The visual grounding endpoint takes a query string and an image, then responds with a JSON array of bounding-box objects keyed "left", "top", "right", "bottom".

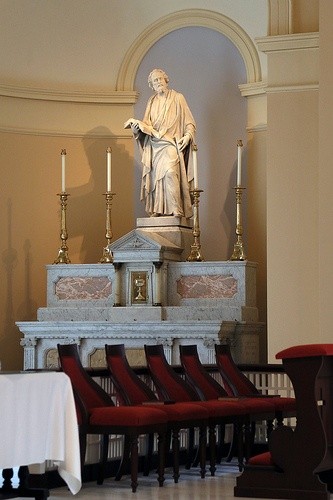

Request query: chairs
[
  {"left": 214, "top": 346, "right": 296, "bottom": 463},
  {"left": 143, "top": 344, "right": 253, "bottom": 476},
  {"left": 179, "top": 345, "right": 278, "bottom": 472},
  {"left": 57, "top": 343, "right": 166, "bottom": 492},
  {"left": 105, "top": 344, "right": 209, "bottom": 483}
]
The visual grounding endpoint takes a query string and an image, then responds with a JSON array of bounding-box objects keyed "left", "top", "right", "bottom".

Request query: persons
[{"left": 124, "top": 69, "right": 196, "bottom": 218}]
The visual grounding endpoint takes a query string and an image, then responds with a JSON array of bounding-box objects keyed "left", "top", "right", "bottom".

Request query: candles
[
  {"left": 60, "top": 149, "right": 67, "bottom": 192},
  {"left": 192, "top": 145, "right": 199, "bottom": 190},
  {"left": 236, "top": 139, "right": 243, "bottom": 186},
  {"left": 106, "top": 147, "right": 113, "bottom": 192}
]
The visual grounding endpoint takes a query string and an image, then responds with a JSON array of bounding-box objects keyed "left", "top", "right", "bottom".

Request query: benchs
[{"left": 234, "top": 344, "right": 333, "bottom": 500}]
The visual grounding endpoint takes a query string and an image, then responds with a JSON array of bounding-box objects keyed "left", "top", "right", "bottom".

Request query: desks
[
  {"left": 0, "top": 374, "right": 81, "bottom": 495},
  {"left": 13, "top": 318, "right": 237, "bottom": 375}
]
